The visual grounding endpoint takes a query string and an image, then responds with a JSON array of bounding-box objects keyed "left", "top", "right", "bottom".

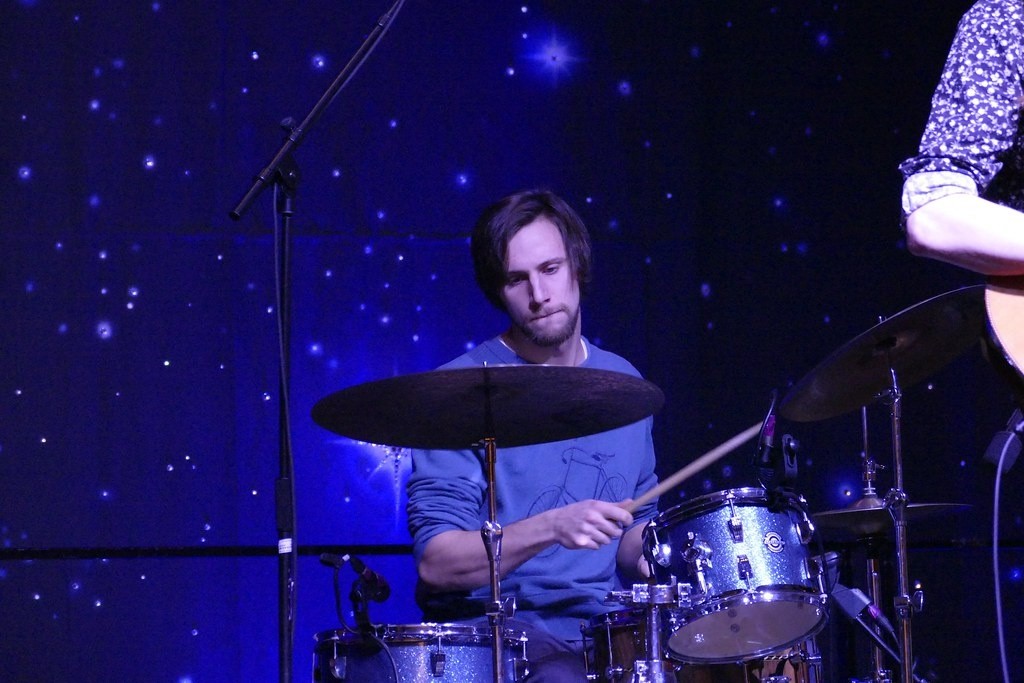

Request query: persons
[
  {"left": 897, "top": 0, "right": 1024, "bottom": 284},
  {"left": 406, "top": 188, "right": 661, "bottom": 683}
]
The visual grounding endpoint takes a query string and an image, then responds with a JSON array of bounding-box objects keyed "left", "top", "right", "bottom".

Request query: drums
[
  {"left": 642, "top": 486, "right": 825, "bottom": 664},
  {"left": 576, "top": 606, "right": 713, "bottom": 683},
  {"left": 313, "top": 623, "right": 529, "bottom": 683}
]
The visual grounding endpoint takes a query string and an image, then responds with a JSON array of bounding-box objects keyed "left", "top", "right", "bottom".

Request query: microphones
[
  {"left": 754, "top": 393, "right": 778, "bottom": 488},
  {"left": 351, "top": 555, "right": 391, "bottom": 602}
]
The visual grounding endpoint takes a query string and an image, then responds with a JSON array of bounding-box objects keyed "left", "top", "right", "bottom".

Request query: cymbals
[
  {"left": 779, "top": 284, "right": 985, "bottom": 423},
  {"left": 312, "top": 364, "right": 665, "bottom": 452},
  {"left": 809, "top": 498, "right": 966, "bottom": 535}
]
